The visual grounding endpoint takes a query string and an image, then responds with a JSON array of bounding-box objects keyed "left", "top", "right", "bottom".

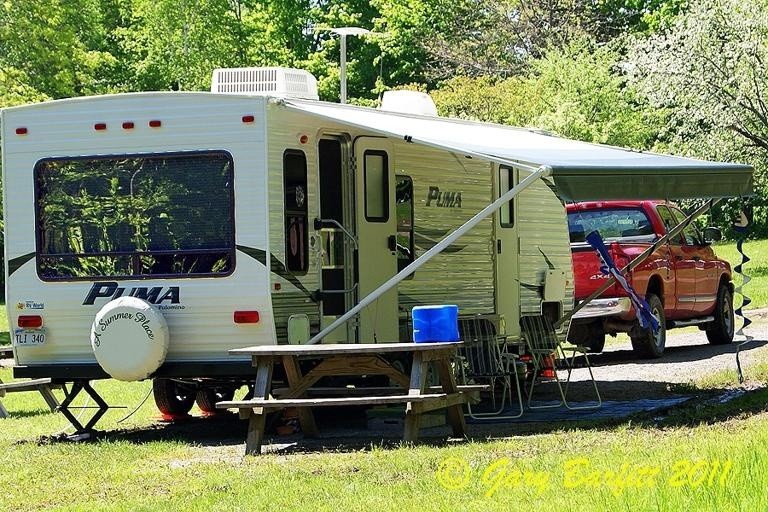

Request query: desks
[{"left": 229, "top": 340, "right": 481, "bottom": 456}]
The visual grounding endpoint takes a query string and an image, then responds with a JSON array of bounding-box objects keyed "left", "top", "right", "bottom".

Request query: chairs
[
  {"left": 569, "top": 224, "right": 585, "bottom": 242},
  {"left": 622, "top": 229, "right": 643, "bottom": 237},
  {"left": 519, "top": 315, "right": 602, "bottom": 411},
  {"left": 456, "top": 318, "right": 524, "bottom": 420}
]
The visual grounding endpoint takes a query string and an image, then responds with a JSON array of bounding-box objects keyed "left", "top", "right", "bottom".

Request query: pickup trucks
[{"left": 566, "top": 200, "right": 735, "bottom": 358}]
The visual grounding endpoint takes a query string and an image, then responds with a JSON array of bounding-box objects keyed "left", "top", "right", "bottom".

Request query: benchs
[
  {"left": 214, "top": 393, "right": 446, "bottom": 410},
  {"left": 272, "top": 384, "right": 492, "bottom": 398},
  {"left": 0, "top": 378, "right": 61, "bottom": 418}
]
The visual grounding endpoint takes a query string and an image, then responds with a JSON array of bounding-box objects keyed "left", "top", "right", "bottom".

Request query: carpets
[{"left": 460, "top": 396, "right": 695, "bottom": 424}]
